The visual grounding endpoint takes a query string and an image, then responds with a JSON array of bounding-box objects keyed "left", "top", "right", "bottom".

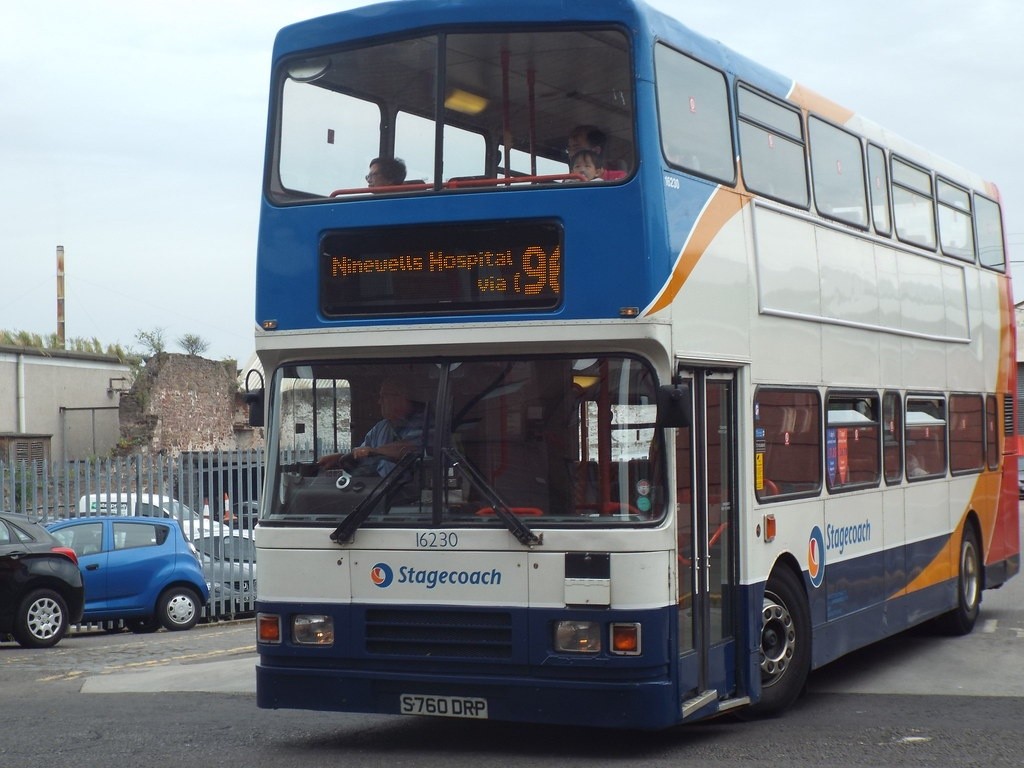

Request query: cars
[
  {"left": 1, "top": 509, "right": 85, "bottom": 649},
  {"left": 215, "top": 501, "right": 260, "bottom": 527},
  {"left": 32, "top": 515, "right": 210, "bottom": 633},
  {"left": 187, "top": 528, "right": 256, "bottom": 614}
]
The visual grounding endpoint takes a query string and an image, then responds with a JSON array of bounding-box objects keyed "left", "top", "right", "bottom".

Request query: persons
[
  {"left": 562, "top": 124, "right": 629, "bottom": 183},
  {"left": 316, "top": 375, "right": 435, "bottom": 507},
  {"left": 365, "top": 158, "right": 406, "bottom": 195},
  {"left": 906, "top": 452, "right": 930, "bottom": 477}
]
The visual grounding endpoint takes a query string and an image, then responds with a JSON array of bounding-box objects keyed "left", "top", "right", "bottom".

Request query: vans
[{"left": 78, "top": 493, "right": 231, "bottom": 553}]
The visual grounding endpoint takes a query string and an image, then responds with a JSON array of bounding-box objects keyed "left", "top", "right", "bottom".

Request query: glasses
[
  {"left": 365, "top": 171, "right": 383, "bottom": 180},
  {"left": 565, "top": 145, "right": 593, "bottom": 154}
]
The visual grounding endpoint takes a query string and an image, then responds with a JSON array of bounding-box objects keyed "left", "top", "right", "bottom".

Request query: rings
[{"left": 361, "top": 450, "right": 365, "bottom": 452}]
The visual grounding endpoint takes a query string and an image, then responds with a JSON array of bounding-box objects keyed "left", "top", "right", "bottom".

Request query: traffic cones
[
  {"left": 225, "top": 492, "right": 238, "bottom": 524},
  {"left": 203, "top": 497, "right": 211, "bottom": 518}
]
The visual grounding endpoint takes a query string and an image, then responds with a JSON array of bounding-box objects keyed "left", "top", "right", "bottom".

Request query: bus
[{"left": 240, "top": 0, "right": 1022, "bottom": 737}]
